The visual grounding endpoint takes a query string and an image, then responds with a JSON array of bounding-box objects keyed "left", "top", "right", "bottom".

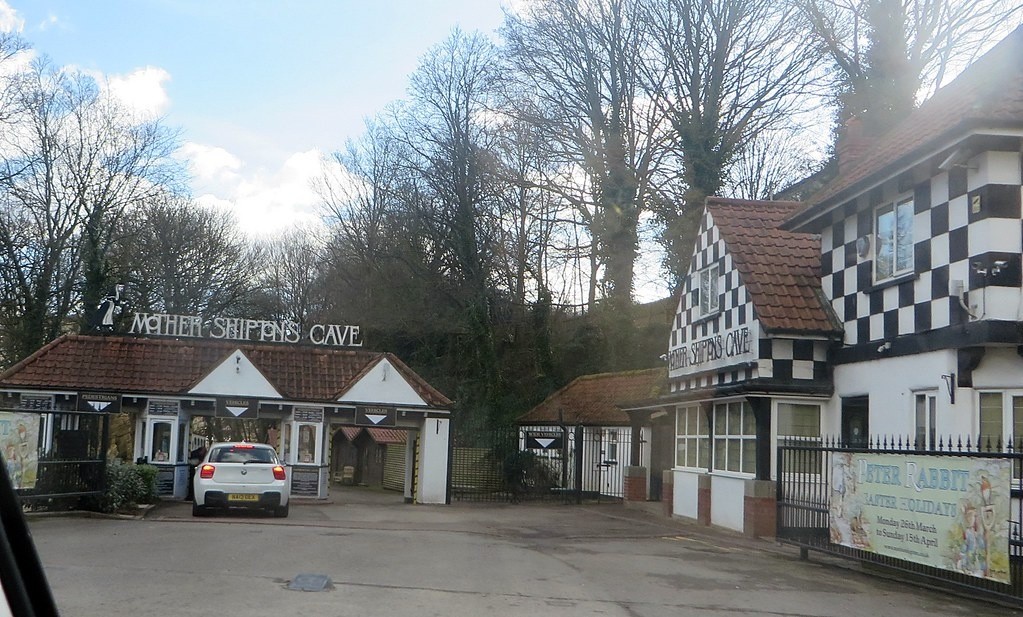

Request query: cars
[{"left": 191, "top": 442, "right": 290, "bottom": 519}]
